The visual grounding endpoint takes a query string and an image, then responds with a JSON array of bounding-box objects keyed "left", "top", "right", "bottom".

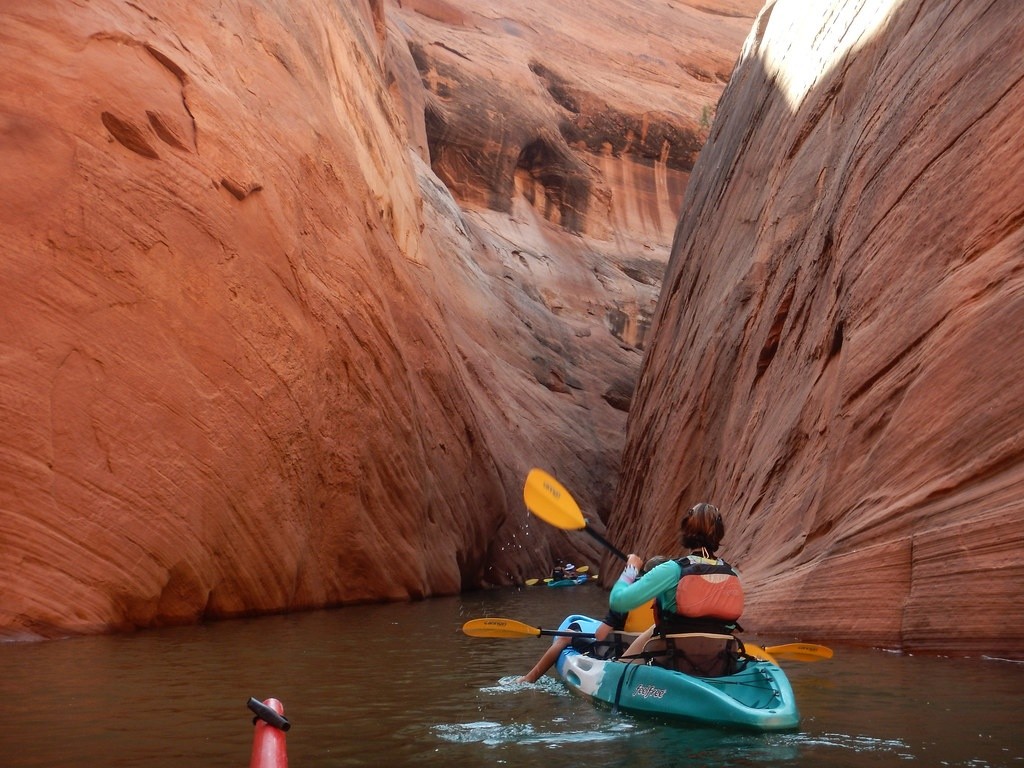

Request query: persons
[
  {"left": 513, "top": 502, "right": 747, "bottom": 685},
  {"left": 565, "top": 563, "right": 576, "bottom": 579},
  {"left": 550, "top": 557, "right": 570, "bottom": 579}
]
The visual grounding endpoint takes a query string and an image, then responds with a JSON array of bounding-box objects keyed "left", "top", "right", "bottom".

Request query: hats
[
  {"left": 644, "top": 555, "right": 668, "bottom": 573},
  {"left": 554, "top": 558, "right": 563, "bottom": 567},
  {"left": 564, "top": 563, "right": 574, "bottom": 571}
]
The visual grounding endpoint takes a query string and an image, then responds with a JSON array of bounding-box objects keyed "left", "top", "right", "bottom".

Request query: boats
[
  {"left": 546, "top": 573, "right": 589, "bottom": 588},
  {"left": 552, "top": 615, "right": 803, "bottom": 729}
]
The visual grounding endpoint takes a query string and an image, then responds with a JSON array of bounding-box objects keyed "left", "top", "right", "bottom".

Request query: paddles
[
  {"left": 524, "top": 466, "right": 782, "bottom": 671},
  {"left": 525, "top": 565, "right": 599, "bottom": 586},
  {"left": 462, "top": 617, "right": 834, "bottom": 663}
]
